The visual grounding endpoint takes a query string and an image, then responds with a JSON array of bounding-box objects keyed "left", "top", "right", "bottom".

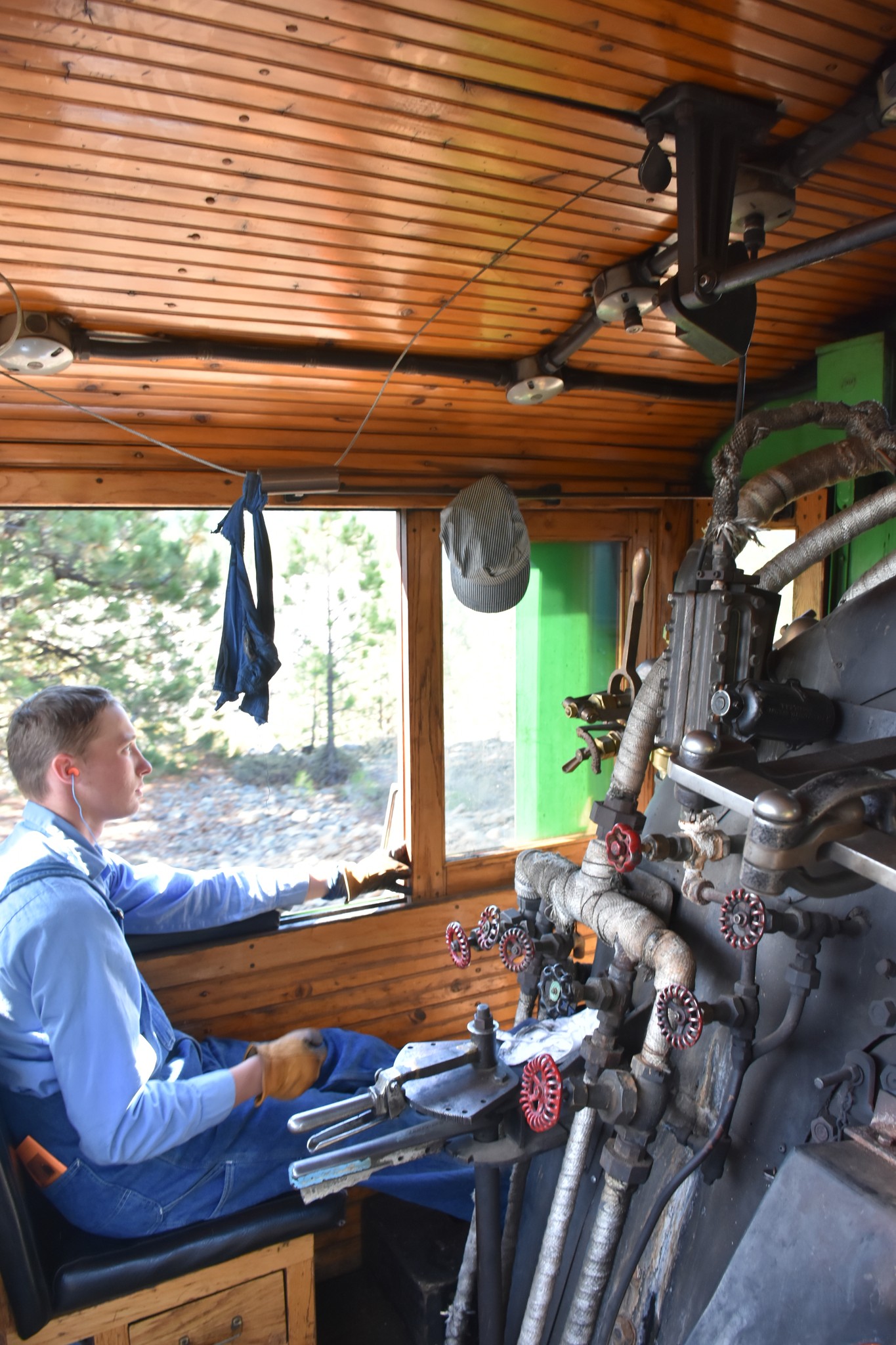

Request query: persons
[{"left": 0, "top": 687, "right": 508, "bottom": 1236}]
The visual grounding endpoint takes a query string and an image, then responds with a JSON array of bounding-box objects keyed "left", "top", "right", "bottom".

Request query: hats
[{"left": 439, "top": 472, "right": 532, "bottom": 615}]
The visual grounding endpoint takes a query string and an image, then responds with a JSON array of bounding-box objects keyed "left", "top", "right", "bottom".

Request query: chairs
[{"left": 0, "top": 1118, "right": 346, "bottom": 1345}]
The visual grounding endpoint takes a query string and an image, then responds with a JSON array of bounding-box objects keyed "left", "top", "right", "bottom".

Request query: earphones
[{"left": 68, "top": 768, "right": 79, "bottom": 776}]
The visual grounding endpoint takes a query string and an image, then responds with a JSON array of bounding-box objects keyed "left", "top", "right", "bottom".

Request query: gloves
[
  {"left": 341, "top": 840, "right": 413, "bottom": 905},
  {"left": 243, "top": 1027, "right": 328, "bottom": 1109}
]
